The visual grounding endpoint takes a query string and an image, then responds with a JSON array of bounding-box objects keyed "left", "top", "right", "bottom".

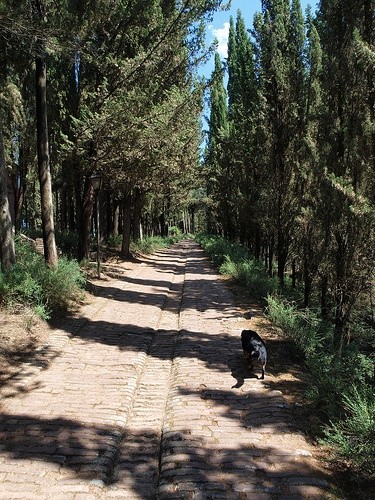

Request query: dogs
[{"left": 240, "top": 329, "right": 266, "bottom": 380}]
